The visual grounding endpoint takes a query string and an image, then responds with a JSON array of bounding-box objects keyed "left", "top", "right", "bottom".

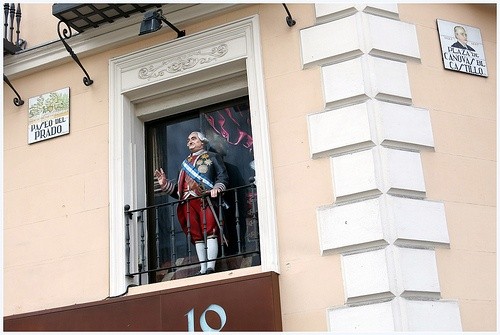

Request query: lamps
[{"left": 137, "top": 8, "right": 186, "bottom": 38}]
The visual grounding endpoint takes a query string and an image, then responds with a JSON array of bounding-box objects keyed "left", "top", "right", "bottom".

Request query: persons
[
  {"left": 451, "top": 26, "right": 475, "bottom": 51},
  {"left": 154, "top": 132, "right": 229, "bottom": 275}
]
